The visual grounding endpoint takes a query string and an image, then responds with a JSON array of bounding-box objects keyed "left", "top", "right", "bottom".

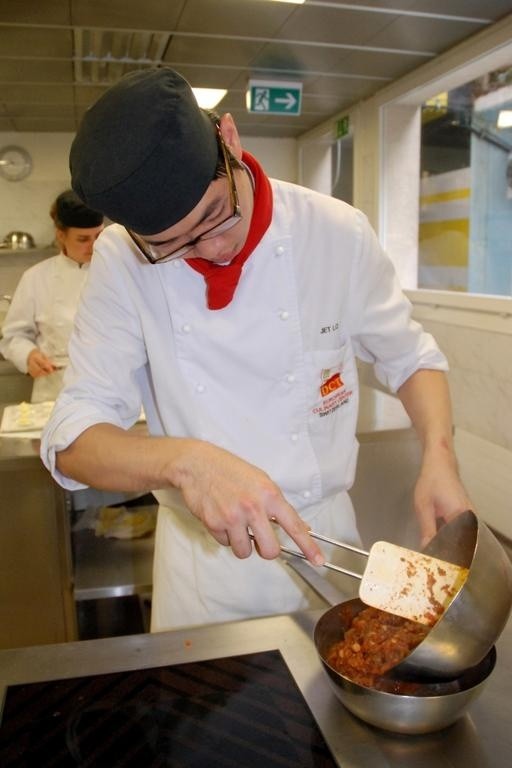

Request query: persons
[
  {"left": 0, "top": 190, "right": 105, "bottom": 403},
  {"left": 40, "top": 66, "right": 476, "bottom": 634}
]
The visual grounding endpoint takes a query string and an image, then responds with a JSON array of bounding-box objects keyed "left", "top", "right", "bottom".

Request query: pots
[
  {"left": 398, "top": 510, "right": 510, "bottom": 677},
  {"left": 286, "top": 555, "right": 497, "bottom": 735}
]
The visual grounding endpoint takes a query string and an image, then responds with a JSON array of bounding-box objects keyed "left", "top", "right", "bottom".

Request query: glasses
[{"left": 125, "top": 122, "right": 243, "bottom": 264}]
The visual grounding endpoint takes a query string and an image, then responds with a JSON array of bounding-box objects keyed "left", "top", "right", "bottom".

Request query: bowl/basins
[{"left": 5, "top": 233, "right": 36, "bottom": 246}]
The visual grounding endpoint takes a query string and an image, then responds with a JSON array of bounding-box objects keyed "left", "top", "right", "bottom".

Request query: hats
[
  {"left": 54, "top": 190, "right": 104, "bottom": 228},
  {"left": 68, "top": 63, "right": 219, "bottom": 236}
]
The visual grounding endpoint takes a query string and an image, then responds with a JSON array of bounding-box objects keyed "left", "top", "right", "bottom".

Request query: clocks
[{"left": 0, "top": 145, "right": 31, "bottom": 182}]
